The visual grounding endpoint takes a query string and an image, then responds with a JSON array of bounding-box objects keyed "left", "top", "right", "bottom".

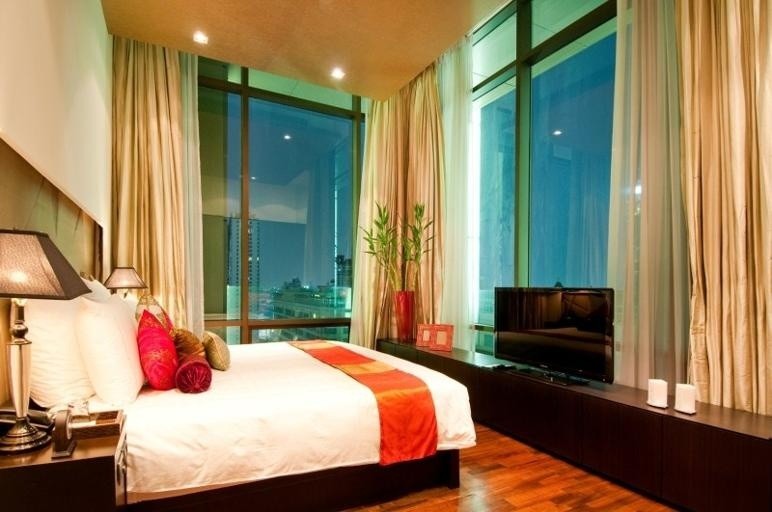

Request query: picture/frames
[
  {"left": 428, "top": 324, "right": 455, "bottom": 353},
  {"left": 415, "top": 321, "right": 432, "bottom": 346}
]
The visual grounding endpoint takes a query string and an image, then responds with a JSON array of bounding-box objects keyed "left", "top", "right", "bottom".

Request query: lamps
[
  {"left": 103, "top": 266, "right": 148, "bottom": 300},
  {"left": 0, "top": 223, "right": 92, "bottom": 452}
]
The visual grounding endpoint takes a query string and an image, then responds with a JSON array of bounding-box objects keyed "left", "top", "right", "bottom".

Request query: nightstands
[{"left": 1, "top": 412, "right": 132, "bottom": 512}]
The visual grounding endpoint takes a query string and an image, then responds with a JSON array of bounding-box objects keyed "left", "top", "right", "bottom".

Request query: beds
[{"left": 1, "top": 327, "right": 478, "bottom": 511}]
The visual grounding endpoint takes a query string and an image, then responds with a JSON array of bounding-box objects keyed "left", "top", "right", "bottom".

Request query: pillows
[{"left": 22, "top": 276, "right": 231, "bottom": 411}]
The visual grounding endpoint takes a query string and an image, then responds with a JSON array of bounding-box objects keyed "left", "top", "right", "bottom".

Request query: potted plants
[{"left": 358, "top": 198, "right": 438, "bottom": 344}]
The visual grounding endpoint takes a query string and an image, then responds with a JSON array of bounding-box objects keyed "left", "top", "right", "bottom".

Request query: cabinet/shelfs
[{"left": 371, "top": 335, "right": 772, "bottom": 512}]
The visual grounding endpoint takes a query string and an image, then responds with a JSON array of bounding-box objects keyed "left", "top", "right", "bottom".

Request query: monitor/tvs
[{"left": 494, "top": 287, "right": 614, "bottom": 387}]
